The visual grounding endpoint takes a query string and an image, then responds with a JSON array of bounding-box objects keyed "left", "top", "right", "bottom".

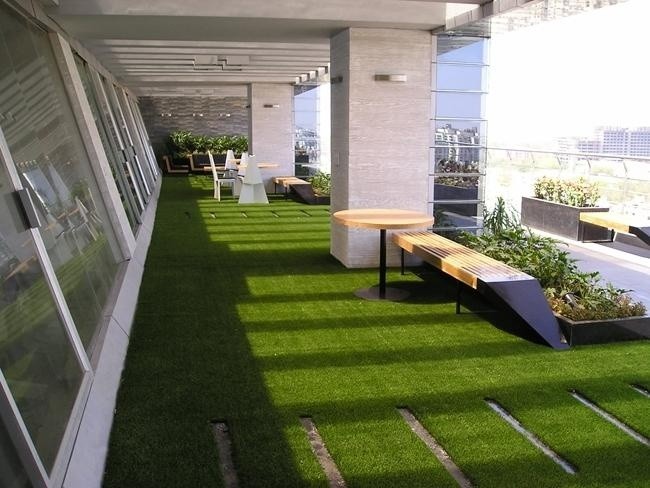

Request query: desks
[
  {"left": 233, "top": 162, "right": 280, "bottom": 190},
  {"left": 331, "top": 207, "right": 435, "bottom": 303}
]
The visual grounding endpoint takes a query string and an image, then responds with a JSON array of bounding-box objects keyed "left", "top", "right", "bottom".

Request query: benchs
[
  {"left": 392, "top": 230, "right": 570, "bottom": 354},
  {"left": 272, "top": 176, "right": 313, "bottom": 204}
]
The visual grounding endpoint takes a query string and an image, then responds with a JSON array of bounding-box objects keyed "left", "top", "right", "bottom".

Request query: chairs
[{"left": 162, "top": 149, "right": 239, "bottom": 202}]
